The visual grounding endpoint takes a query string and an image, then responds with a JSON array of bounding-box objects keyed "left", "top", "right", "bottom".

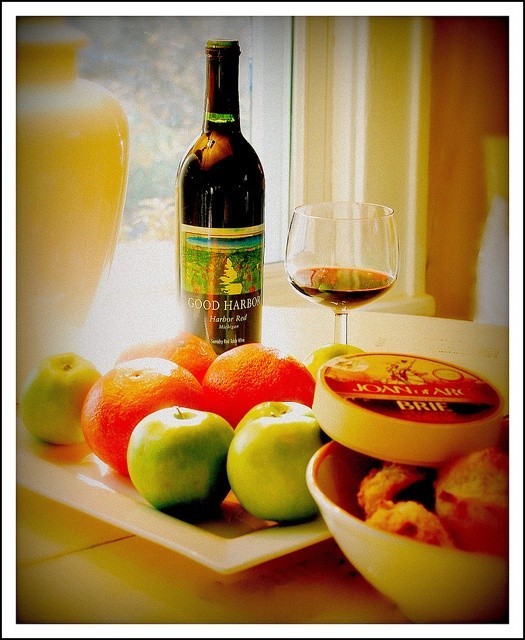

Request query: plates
[{"left": 16, "top": 304, "right": 508, "bottom": 575}]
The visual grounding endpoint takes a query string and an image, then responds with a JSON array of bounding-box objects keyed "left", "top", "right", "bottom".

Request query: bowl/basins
[{"left": 304, "top": 437, "right": 509, "bottom": 623}]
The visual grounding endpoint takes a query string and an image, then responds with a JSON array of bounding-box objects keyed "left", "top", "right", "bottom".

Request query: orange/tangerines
[
  {"left": 205, "top": 340, "right": 317, "bottom": 427},
  {"left": 80, "top": 357, "right": 205, "bottom": 475},
  {"left": 110, "top": 329, "right": 215, "bottom": 379}
]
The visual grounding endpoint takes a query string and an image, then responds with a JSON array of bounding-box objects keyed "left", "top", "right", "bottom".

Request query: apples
[
  {"left": 125, "top": 404, "right": 240, "bottom": 514},
  {"left": 222, "top": 398, "right": 325, "bottom": 519},
  {"left": 19, "top": 349, "right": 104, "bottom": 444}
]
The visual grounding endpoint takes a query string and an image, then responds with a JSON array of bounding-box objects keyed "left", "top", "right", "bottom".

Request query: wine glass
[{"left": 285, "top": 202, "right": 399, "bottom": 345}]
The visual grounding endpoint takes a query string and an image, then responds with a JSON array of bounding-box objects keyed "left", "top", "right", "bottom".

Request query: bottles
[{"left": 176, "top": 38, "right": 265, "bottom": 354}]
